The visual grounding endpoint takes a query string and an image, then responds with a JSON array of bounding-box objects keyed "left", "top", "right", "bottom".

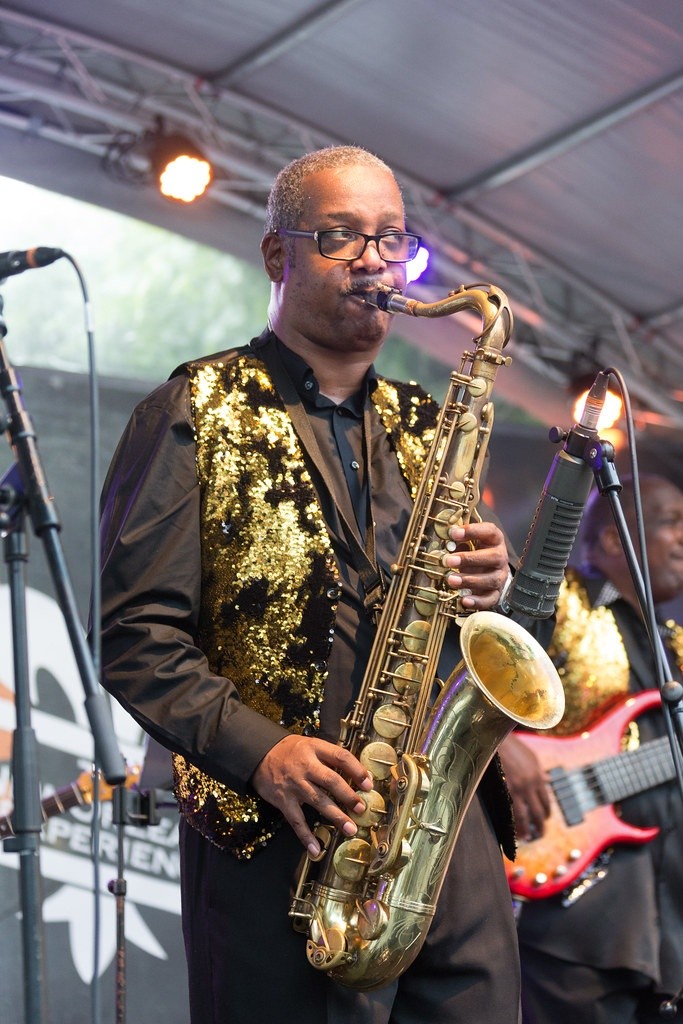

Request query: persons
[
  {"left": 92, "top": 142, "right": 523, "bottom": 1024},
  {"left": 512, "top": 422, "right": 682, "bottom": 1022}
]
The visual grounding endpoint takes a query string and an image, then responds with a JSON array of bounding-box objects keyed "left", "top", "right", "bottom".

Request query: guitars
[
  {"left": 2, "top": 763, "right": 142, "bottom": 840},
  {"left": 493, "top": 689, "right": 682, "bottom": 902}
]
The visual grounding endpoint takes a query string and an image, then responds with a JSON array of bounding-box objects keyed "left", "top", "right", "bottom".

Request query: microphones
[
  {"left": 506, "top": 371, "right": 612, "bottom": 620},
  {"left": 0, "top": 247, "right": 63, "bottom": 280}
]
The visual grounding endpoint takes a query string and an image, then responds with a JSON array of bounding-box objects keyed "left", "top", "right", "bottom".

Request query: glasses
[{"left": 272, "top": 227, "right": 422, "bottom": 263}]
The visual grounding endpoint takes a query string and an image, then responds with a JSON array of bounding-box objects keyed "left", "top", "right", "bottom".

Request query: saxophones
[{"left": 294, "top": 279, "right": 568, "bottom": 986}]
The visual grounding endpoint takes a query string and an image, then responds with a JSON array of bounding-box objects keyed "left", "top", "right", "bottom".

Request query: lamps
[
  {"left": 567, "top": 373, "right": 623, "bottom": 432},
  {"left": 152, "top": 132, "right": 213, "bottom": 205}
]
